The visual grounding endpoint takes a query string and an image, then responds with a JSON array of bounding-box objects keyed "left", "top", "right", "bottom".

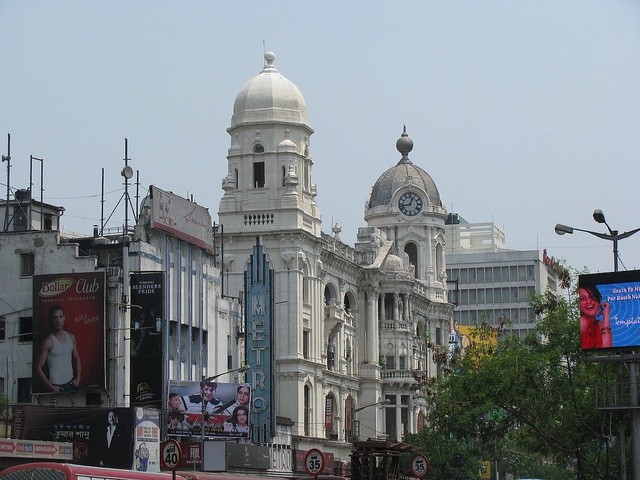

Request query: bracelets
[{"left": 600, "top": 327, "right": 612, "bottom": 335}]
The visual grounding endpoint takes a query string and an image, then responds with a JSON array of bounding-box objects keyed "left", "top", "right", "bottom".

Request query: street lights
[
  {"left": 556, "top": 208, "right": 640, "bottom": 479},
  {"left": 353, "top": 398, "right": 390, "bottom": 440},
  {"left": 200, "top": 365, "right": 250, "bottom": 471}
]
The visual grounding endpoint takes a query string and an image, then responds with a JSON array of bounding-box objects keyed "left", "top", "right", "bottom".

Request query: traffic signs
[
  {"left": 411, "top": 454, "right": 428, "bottom": 478},
  {"left": 161, "top": 439, "right": 182, "bottom": 469},
  {"left": 0, "top": 438, "right": 73, "bottom": 461},
  {"left": 305, "top": 448, "right": 325, "bottom": 476}
]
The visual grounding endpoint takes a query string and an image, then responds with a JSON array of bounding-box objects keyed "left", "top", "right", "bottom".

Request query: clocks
[{"left": 398, "top": 192, "right": 423, "bottom": 217}]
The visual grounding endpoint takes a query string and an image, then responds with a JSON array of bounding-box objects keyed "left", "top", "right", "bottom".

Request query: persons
[
  {"left": 169, "top": 393, "right": 181, "bottom": 412},
  {"left": 179, "top": 382, "right": 223, "bottom": 414},
  {"left": 34, "top": 305, "right": 83, "bottom": 392},
  {"left": 224, "top": 406, "right": 248, "bottom": 432},
  {"left": 579, "top": 285, "right": 613, "bottom": 348},
  {"left": 97, "top": 410, "right": 129, "bottom": 468},
  {"left": 223, "top": 385, "right": 249, "bottom": 414}
]
